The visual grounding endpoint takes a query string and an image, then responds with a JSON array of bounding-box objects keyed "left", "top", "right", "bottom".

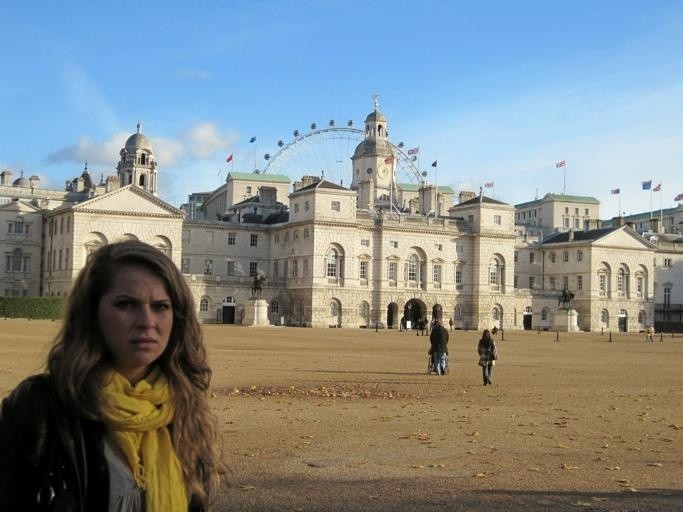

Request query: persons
[
  {"left": 0, "top": 240, "right": 234, "bottom": 511},
  {"left": 644, "top": 324, "right": 656, "bottom": 342},
  {"left": 416, "top": 317, "right": 455, "bottom": 337},
  {"left": 428, "top": 325, "right": 450, "bottom": 376},
  {"left": 477, "top": 328, "right": 496, "bottom": 386}
]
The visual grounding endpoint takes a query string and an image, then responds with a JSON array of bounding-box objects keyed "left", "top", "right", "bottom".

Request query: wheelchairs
[{"left": 426, "top": 352, "right": 450, "bottom": 375}]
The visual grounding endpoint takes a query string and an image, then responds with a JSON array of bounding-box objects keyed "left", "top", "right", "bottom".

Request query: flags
[
  {"left": 609, "top": 187, "right": 620, "bottom": 195},
  {"left": 653, "top": 183, "right": 660, "bottom": 192},
  {"left": 430, "top": 160, "right": 438, "bottom": 168},
  {"left": 226, "top": 154, "right": 234, "bottom": 164},
  {"left": 673, "top": 193, "right": 683, "bottom": 202},
  {"left": 384, "top": 147, "right": 419, "bottom": 165},
  {"left": 483, "top": 180, "right": 493, "bottom": 189},
  {"left": 420, "top": 170, "right": 428, "bottom": 178},
  {"left": 555, "top": 160, "right": 566, "bottom": 169},
  {"left": 640, "top": 178, "right": 652, "bottom": 190},
  {"left": 248, "top": 134, "right": 258, "bottom": 145}
]
